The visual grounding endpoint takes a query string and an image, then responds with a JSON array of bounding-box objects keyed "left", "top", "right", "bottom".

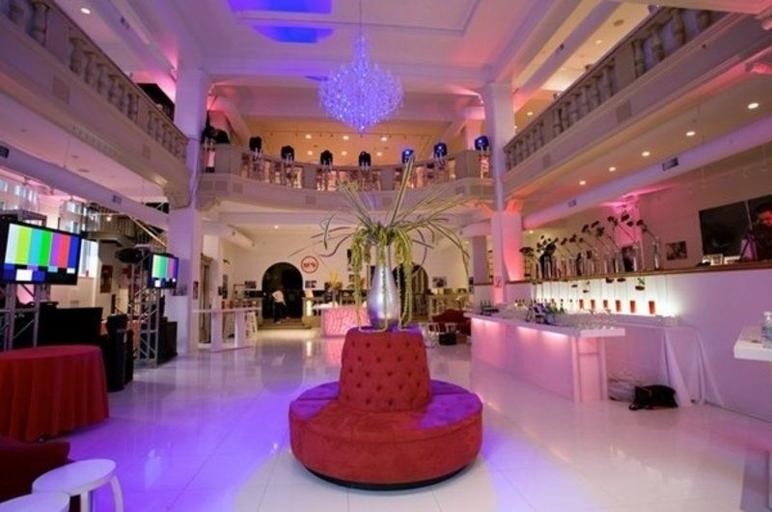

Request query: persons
[
  {"left": 540, "top": 244, "right": 556, "bottom": 278},
  {"left": 755, "top": 198, "right": 772, "bottom": 261},
  {"left": 272, "top": 284, "right": 287, "bottom": 326}
]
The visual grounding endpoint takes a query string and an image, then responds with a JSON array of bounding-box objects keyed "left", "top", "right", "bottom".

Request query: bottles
[
  {"left": 762, "top": 311, "right": 771, "bottom": 347},
  {"left": 478, "top": 297, "right": 564, "bottom": 327}
]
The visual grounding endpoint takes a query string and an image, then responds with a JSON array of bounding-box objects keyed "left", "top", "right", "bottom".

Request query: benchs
[{"left": 287, "top": 324, "right": 483, "bottom": 486}]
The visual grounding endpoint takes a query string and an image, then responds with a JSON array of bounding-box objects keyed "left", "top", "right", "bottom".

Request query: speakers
[
  {"left": 140, "top": 321, "right": 177, "bottom": 367},
  {"left": 8, "top": 308, "right": 103, "bottom": 348}
]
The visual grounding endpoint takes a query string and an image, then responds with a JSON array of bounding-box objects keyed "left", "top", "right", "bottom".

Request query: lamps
[{"left": 315, "top": 0, "right": 405, "bottom": 137}]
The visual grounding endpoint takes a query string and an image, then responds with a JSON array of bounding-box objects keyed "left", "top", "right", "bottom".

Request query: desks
[
  {"left": 0, "top": 343, "right": 110, "bottom": 443},
  {"left": 541, "top": 312, "right": 726, "bottom": 408},
  {"left": 310, "top": 287, "right": 476, "bottom": 346},
  {"left": 189, "top": 306, "right": 262, "bottom": 352},
  {"left": 461, "top": 309, "right": 626, "bottom": 405},
  {"left": 732, "top": 324, "right": 772, "bottom": 510}
]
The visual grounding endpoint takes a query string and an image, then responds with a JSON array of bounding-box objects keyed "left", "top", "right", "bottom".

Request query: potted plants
[{"left": 288, "top": 156, "right": 480, "bottom": 333}]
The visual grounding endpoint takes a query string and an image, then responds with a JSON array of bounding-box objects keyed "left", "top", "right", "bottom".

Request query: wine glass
[{"left": 559, "top": 306, "right": 618, "bottom": 329}]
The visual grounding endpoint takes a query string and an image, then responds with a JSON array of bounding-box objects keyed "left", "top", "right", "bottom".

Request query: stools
[
  {"left": 0, "top": 493, "right": 71, "bottom": 511},
  {"left": 29, "top": 456, "right": 125, "bottom": 512}
]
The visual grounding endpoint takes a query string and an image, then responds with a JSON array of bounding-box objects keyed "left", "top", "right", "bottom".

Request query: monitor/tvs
[
  {"left": 146, "top": 252, "right": 179, "bottom": 289},
  {"left": 0, "top": 220, "right": 81, "bottom": 285}
]
